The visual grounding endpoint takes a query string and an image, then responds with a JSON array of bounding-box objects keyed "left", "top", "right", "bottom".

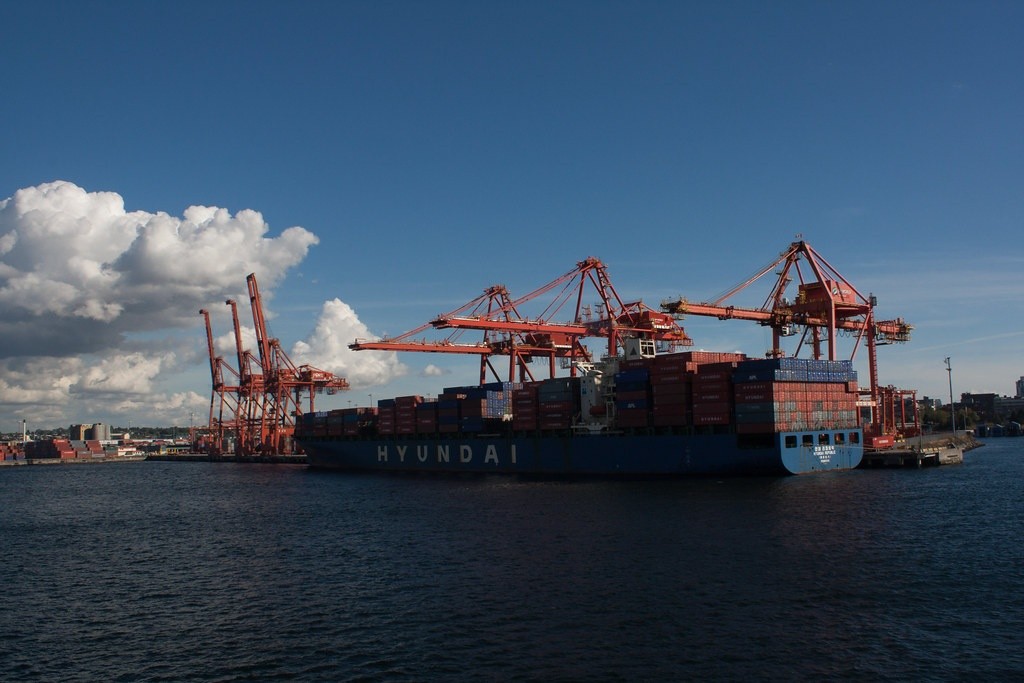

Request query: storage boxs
[
  {"left": 798, "top": 280, "right": 856, "bottom": 304},
  {"left": 634, "top": 311, "right": 666, "bottom": 325},
  {"left": 245, "top": 374, "right": 265, "bottom": 384},
  {"left": 293, "top": 351, "right": 860, "bottom": 437},
  {"left": 525, "top": 333, "right": 566, "bottom": 346},
  {"left": 863, "top": 435, "right": 894, "bottom": 447},
  {"left": 0, "top": 438, "right": 106, "bottom": 462}
]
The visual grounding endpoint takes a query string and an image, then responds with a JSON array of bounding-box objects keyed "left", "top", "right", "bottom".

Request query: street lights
[{"left": 945, "top": 356, "right": 955, "bottom": 436}]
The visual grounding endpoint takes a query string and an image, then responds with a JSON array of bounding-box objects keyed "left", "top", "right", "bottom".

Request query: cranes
[
  {"left": 658, "top": 235, "right": 916, "bottom": 362},
  {"left": 427, "top": 252, "right": 694, "bottom": 358},
  {"left": 347, "top": 282, "right": 593, "bottom": 368},
  {"left": 197, "top": 271, "right": 352, "bottom": 463}
]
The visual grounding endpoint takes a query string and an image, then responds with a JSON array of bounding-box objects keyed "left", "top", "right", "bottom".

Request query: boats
[{"left": 289, "top": 425, "right": 865, "bottom": 477}]
